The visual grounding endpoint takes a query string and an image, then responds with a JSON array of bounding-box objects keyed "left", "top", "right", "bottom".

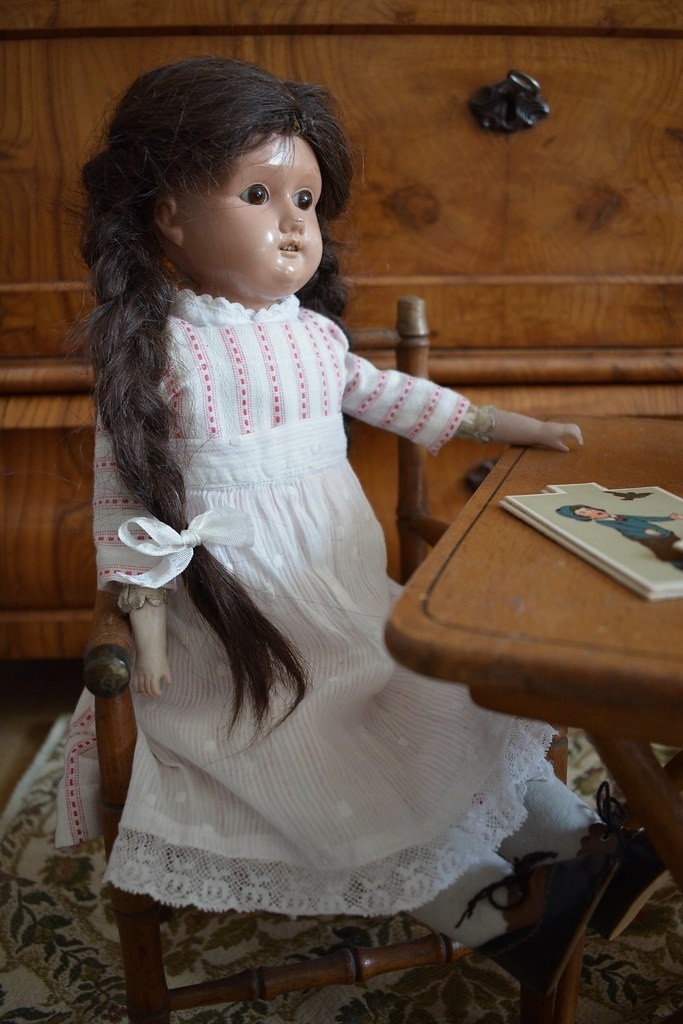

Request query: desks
[{"left": 385, "top": 411, "right": 683, "bottom": 1024}]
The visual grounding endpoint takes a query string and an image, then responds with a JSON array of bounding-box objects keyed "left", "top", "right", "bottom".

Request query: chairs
[{"left": 78, "top": 296, "right": 568, "bottom": 1024}]
